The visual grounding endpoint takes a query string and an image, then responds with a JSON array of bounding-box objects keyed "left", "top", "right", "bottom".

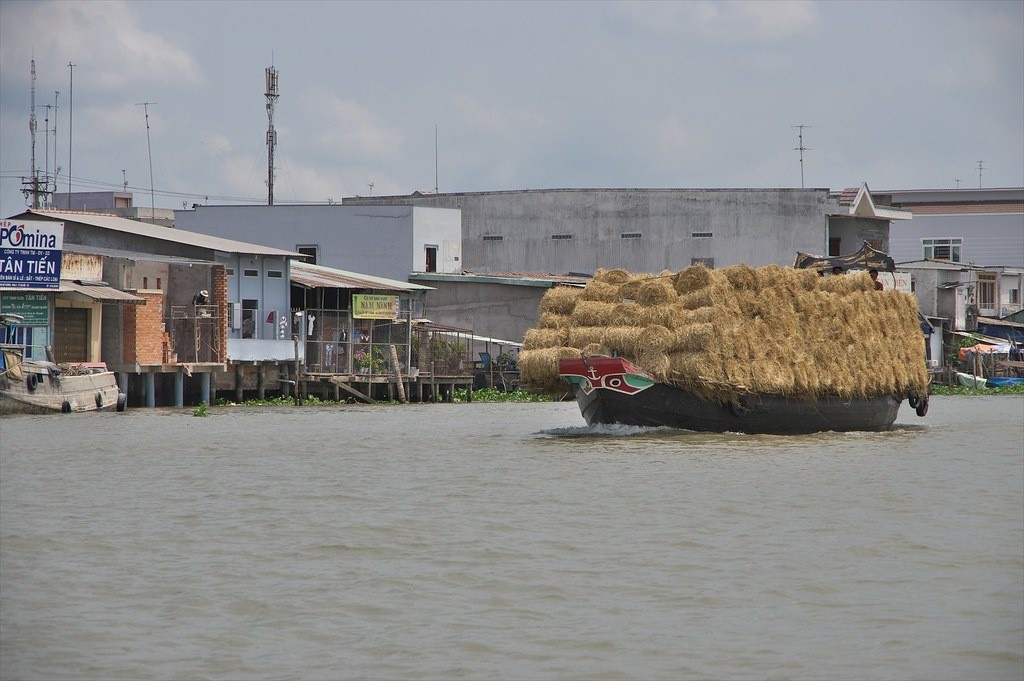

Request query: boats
[
  {"left": 952, "top": 344, "right": 1024, "bottom": 388},
  {"left": 0, "top": 343, "right": 128, "bottom": 413},
  {"left": 559, "top": 239, "right": 935, "bottom": 435}
]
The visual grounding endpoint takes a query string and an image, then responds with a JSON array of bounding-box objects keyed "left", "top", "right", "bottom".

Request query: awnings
[{"left": 60, "top": 280, "right": 146, "bottom": 305}]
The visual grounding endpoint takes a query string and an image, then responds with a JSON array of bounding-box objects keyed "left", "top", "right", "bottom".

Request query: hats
[
  {"left": 869, "top": 269, "right": 879, "bottom": 275},
  {"left": 200, "top": 290, "right": 209, "bottom": 297}
]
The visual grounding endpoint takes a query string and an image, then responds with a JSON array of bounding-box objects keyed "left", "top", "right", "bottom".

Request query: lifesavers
[
  {"left": 908, "top": 389, "right": 928, "bottom": 416},
  {"left": 728, "top": 387, "right": 750, "bottom": 417},
  {"left": 27, "top": 372, "right": 38, "bottom": 390},
  {"left": 117, "top": 392, "right": 127, "bottom": 411},
  {"left": 95, "top": 392, "right": 103, "bottom": 407},
  {"left": 62, "top": 401, "right": 71, "bottom": 414}
]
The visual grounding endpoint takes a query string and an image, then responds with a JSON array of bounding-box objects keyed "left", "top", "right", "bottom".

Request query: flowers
[{"left": 353, "top": 348, "right": 367, "bottom": 363}]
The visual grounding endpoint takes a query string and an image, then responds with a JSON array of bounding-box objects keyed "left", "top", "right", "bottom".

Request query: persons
[
  {"left": 817, "top": 271, "right": 824, "bottom": 277},
  {"left": 192, "top": 290, "right": 209, "bottom": 315},
  {"left": 833, "top": 267, "right": 842, "bottom": 275},
  {"left": 868, "top": 268, "right": 883, "bottom": 290}
]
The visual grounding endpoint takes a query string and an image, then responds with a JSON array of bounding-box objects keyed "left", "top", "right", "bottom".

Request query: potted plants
[
  {"left": 430, "top": 338, "right": 466, "bottom": 375},
  {"left": 497, "top": 354, "right": 516, "bottom": 371},
  {"left": 354, "top": 347, "right": 388, "bottom": 374}
]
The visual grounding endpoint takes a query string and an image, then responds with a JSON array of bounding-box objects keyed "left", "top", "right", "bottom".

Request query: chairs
[{"left": 479, "top": 352, "right": 499, "bottom": 370}]
